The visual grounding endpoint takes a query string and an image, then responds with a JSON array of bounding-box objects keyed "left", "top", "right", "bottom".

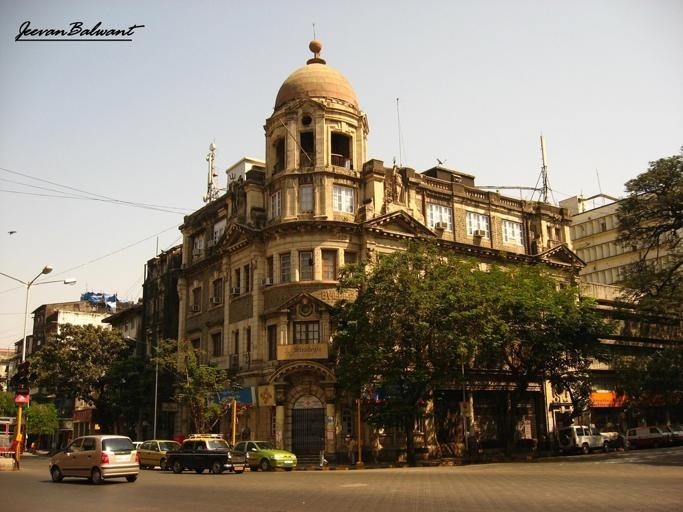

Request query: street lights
[
  {"left": 144, "top": 328, "right": 158, "bottom": 440},
  {"left": 0, "top": 260, "right": 77, "bottom": 361}
]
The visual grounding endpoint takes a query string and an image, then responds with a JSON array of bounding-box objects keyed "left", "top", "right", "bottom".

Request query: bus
[{"left": 0, "top": 415, "right": 27, "bottom": 456}]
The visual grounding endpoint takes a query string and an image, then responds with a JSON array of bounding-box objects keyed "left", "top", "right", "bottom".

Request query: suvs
[
  {"left": 561, "top": 423, "right": 610, "bottom": 455},
  {"left": 164, "top": 430, "right": 246, "bottom": 474}
]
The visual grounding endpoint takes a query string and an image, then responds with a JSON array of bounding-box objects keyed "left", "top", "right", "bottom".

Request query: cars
[
  {"left": 598, "top": 430, "right": 621, "bottom": 442},
  {"left": 137, "top": 440, "right": 180, "bottom": 468},
  {"left": 623, "top": 423, "right": 682, "bottom": 449},
  {"left": 45, "top": 432, "right": 138, "bottom": 485},
  {"left": 231, "top": 439, "right": 299, "bottom": 471},
  {"left": 130, "top": 437, "right": 144, "bottom": 449}
]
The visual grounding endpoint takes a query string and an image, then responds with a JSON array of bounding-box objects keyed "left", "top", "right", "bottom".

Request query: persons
[
  {"left": 391, "top": 165, "right": 403, "bottom": 205},
  {"left": 344, "top": 434, "right": 357, "bottom": 466},
  {"left": 371, "top": 435, "right": 382, "bottom": 465},
  {"left": 318, "top": 434, "right": 329, "bottom": 467}
]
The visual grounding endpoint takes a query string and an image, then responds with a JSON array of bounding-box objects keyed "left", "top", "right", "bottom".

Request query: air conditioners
[
  {"left": 208, "top": 239, "right": 217, "bottom": 247},
  {"left": 188, "top": 304, "right": 201, "bottom": 312},
  {"left": 435, "top": 222, "right": 447, "bottom": 230},
  {"left": 473, "top": 230, "right": 485, "bottom": 238},
  {"left": 259, "top": 277, "right": 272, "bottom": 286},
  {"left": 191, "top": 248, "right": 202, "bottom": 255},
  {"left": 229, "top": 286, "right": 240, "bottom": 295},
  {"left": 208, "top": 296, "right": 222, "bottom": 304}
]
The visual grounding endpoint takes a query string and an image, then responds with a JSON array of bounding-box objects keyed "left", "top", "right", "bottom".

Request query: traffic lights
[{"left": 19, "top": 358, "right": 28, "bottom": 387}]
